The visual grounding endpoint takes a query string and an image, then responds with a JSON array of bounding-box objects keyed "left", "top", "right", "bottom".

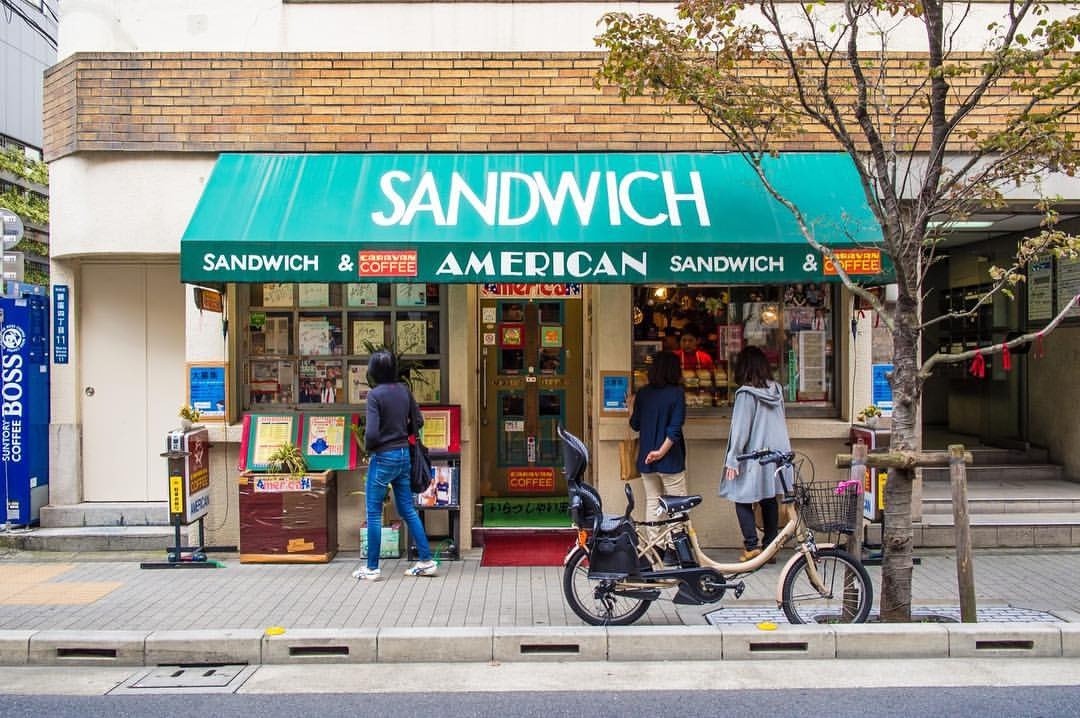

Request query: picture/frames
[{"left": 185, "top": 361, "right": 227, "bottom": 423}]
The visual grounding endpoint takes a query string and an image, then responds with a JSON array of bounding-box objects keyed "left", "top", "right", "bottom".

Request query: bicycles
[{"left": 555, "top": 423, "right": 874, "bottom": 625}]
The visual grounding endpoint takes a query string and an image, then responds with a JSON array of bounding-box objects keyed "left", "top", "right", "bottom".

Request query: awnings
[{"left": 181, "top": 153, "right": 899, "bottom": 284}]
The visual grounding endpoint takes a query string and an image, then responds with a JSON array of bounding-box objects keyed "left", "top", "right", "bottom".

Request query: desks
[{"left": 239, "top": 471, "right": 338, "bottom": 563}]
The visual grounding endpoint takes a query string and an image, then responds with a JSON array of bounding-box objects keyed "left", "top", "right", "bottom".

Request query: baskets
[{"left": 792, "top": 479, "right": 859, "bottom": 534}]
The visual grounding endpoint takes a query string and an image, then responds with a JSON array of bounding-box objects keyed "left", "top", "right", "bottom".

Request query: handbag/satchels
[
  {"left": 618, "top": 437, "right": 641, "bottom": 480},
  {"left": 408, "top": 438, "right": 432, "bottom": 494}
]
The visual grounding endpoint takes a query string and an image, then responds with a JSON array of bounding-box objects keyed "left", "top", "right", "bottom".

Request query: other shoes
[
  {"left": 737, "top": 549, "right": 760, "bottom": 563},
  {"left": 767, "top": 554, "right": 777, "bottom": 564}
]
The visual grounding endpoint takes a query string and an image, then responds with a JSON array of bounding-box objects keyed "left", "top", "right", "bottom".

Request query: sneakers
[
  {"left": 352, "top": 566, "right": 381, "bottom": 581},
  {"left": 404, "top": 561, "right": 437, "bottom": 576}
]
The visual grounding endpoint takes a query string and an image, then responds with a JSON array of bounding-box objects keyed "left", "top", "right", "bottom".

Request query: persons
[
  {"left": 624, "top": 350, "right": 686, "bottom": 566},
  {"left": 718, "top": 345, "right": 795, "bottom": 564},
  {"left": 300, "top": 380, "right": 311, "bottom": 401},
  {"left": 351, "top": 349, "right": 438, "bottom": 579},
  {"left": 309, "top": 381, "right": 322, "bottom": 402},
  {"left": 791, "top": 311, "right": 799, "bottom": 330},
  {"left": 322, "top": 379, "right": 336, "bottom": 404},
  {"left": 811, "top": 310, "right": 823, "bottom": 330},
  {"left": 673, "top": 323, "right": 714, "bottom": 370}
]
